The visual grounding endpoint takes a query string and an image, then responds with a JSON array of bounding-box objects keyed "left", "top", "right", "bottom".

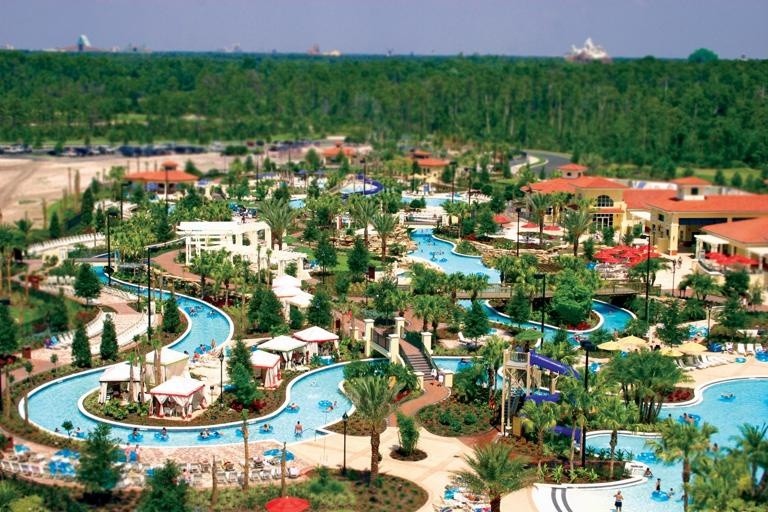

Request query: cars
[
  {"left": 0, "top": 140, "right": 229, "bottom": 160},
  {"left": 266, "top": 136, "right": 322, "bottom": 155}
]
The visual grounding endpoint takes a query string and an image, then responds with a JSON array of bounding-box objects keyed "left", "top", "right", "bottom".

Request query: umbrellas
[{"left": 596, "top": 334, "right": 710, "bottom": 358}]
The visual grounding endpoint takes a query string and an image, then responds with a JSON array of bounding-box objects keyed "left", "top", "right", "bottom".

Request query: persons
[
  {"left": 643, "top": 466, "right": 655, "bottom": 479},
  {"left": 133, "top": 444, "right": 143, "bottom": 464},
  {"left": 76, "top": 426, "right": 80, "bottom": 431},
  {"left": 720, "top": 392, "right": 734, "bottom": 398},
  {"left": 682, "top": 413, "right": 689, "bottom": 422},
  {"left": 200, "top": 430, "right": 209, "bottom": 439},
  {"left": 161, "top": 427, "right": 167, "bottom": 437},
  {"left": 181, "top": 468, "right": 191, "bottom": 483},
  {"left": 333, "top": 401, "right": 338, "bottom": 408},
  {"left": 124, "top": 442, "right": 132, "bottom": 463},
  {"left": 417, "top": 227, "right": 448, "bottom": 262},
  {"left": 263, "top": 423, "right": 271, "bottom": 431},
  {"left": 55, "top": 428, "right": 60, "bottom": 432},
  {"left": 432, "top": 494, "right": 465, "bottom": 509},
  {"left": 10, "top": 451, "right": 31, "bottom": 463},
  {"left": 247, "top": 453, "right": 279, "bottom": 462},
  {"left": 726, "top": 341, "right": 734, "bottom": 353},
  {"left": 325, "top": 405, "right": 334, "bottom": 412},
  {"left": 183, "top": 336, "right": 215, "bottom": 364},
  {"left": 668, "top": 487, "right": 674, "bottom": 498},
  {"left": 688, "top": 415, "right": 694, "bottom": 426},
  {"left": 289, "top": 401, "right": 300, "bottom": 411},
  {"left": 655, "top": 477, "right": 661, "bottom": 491},
  {"left": 712, "top": 442, "right": 720, "bottom": 451},
  {"left": 675, "top": 492, "right": 685, "bottom": 503},
  {"left": 175, "top": 295, "right": 216, "bottom": 318},
  {"left": 205, "top": 428, "right": 225, "bottom": 436},
  {"left": 132, "top": 427, "right": 139, "bottom": 436},
  {"left": 613, "top": 489, "right": 624, "bottom": 512},
  {"left": 295, "top": 420, "right": 304, "bottom": 435}
]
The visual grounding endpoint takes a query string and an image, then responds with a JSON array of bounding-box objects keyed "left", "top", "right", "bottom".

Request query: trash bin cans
[{"left": 22, "top": 345, "right": 31, "bottom": 359}]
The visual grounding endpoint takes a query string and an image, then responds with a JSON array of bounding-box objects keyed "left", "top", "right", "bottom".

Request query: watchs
[{"left": 222, "top": 456, "right": 232, "bottom": 467}]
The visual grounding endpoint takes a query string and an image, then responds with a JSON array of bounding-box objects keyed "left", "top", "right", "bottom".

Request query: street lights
[
  {"left": 217, "top": 350, "right": 227, "bottom": 409},
  {"left": 352, "top": 146, "right": 473, "bottom": 234},
  {"left": 147, "top": 246, "right": 161, "bottom": 341},
  {"left": 319, "top": 251, "right": 325, "bottom": 284},
  {"left": 315, "top": 298, "right": 321, "bottom": 324},
  {"left": 365, "top": 269, "right": 370, "bottom": 309},
  {"left": 342, "top": 411, "right": 352, "bottom": 476},
  {"left": 512, "top": 206, "right": 523, "bottom": 254},
  {"left": 119, "top": 177, "right": 133, "bottom": 223},
  {"left": 576, "top": 338, "right": 594, "bottom": 469},
  {"left": 532, "top": 272, "right": 545, "bottom": 352},
  {"left": 672, "top": 258, "right": 676, "bottom": 298},
  {"left": 707, "top": 305, "right": 713, "bottom": 351},
  {"left": 638, "top": 234, "right": 651, "bottom": 323},
  {"left": 106, "top": 211, "right": 118, "bottom": 287},
  {"left": 162, "top": 164, "right": 175, "bottom": 223}
]
